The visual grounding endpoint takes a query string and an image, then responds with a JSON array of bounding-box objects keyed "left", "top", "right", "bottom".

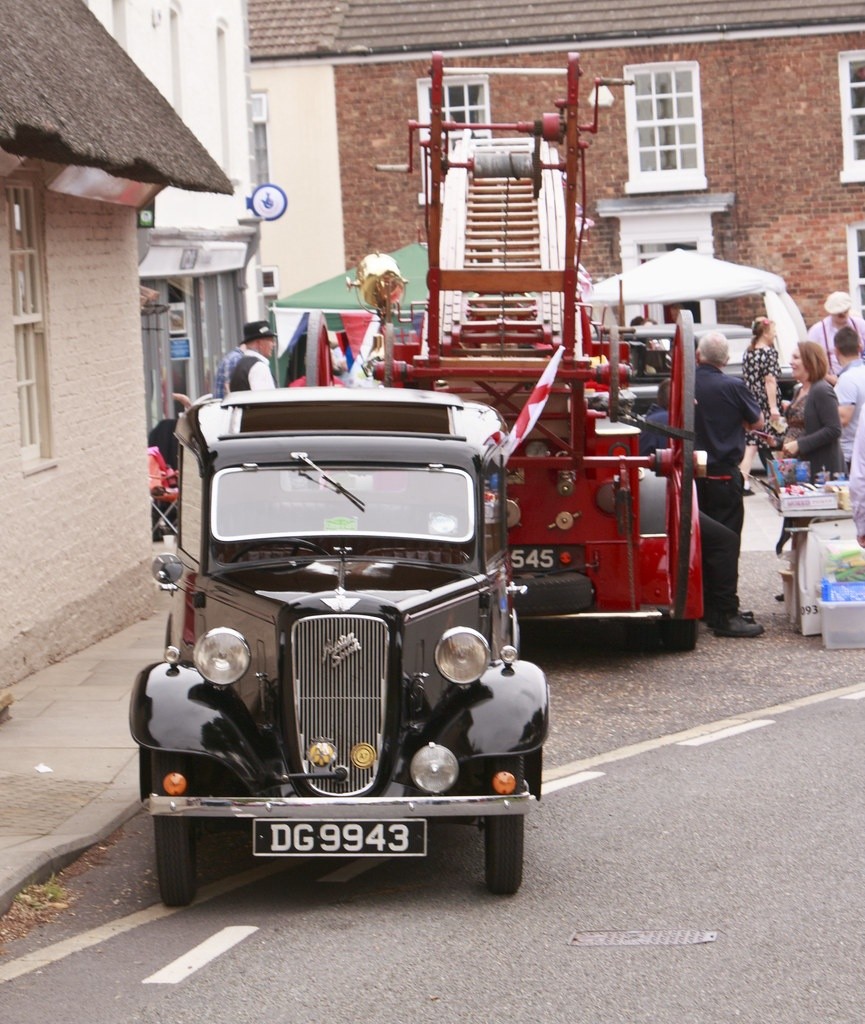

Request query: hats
[
  {"left": 239, "top": 320, "right": 278, "bottom": 345},
  {"left": 823, "top": 290, "right": 853, "bottom": 315}
]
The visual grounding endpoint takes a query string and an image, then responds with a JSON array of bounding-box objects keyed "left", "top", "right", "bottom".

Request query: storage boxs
[
  {"left": 815, "top": 595, "right": 864, "bottom": 650},
  {"left": 768, "top": 485, "right": 838, "bottom": 511}
]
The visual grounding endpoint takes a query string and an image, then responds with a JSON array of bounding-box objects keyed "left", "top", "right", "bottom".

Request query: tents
[
  {"left": 268, "top": 241, "right": 535, "bottom": 388},
  {"left": 585, "top": 247, "right": 807, "bottom": 375}
]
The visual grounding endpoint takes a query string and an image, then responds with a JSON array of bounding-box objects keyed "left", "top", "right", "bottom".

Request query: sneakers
[{"left": 706, "top": 610, "right": 766, "bottom": 638}]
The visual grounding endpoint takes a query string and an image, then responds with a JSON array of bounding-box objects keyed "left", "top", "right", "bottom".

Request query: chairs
[{"left": 146, "top": 446, "right": 179, "bottom": 534}]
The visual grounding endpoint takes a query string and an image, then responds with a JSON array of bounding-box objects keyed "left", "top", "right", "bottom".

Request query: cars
[
  {"left": 128, "top": 387, "right": 550, "bottom": 906},
  {"left": 601, "top": 323, "right": 798, "bottom": 415}
]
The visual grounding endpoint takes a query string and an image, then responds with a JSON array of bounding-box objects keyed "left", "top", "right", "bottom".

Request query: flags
[{"left": 490, "top": 346, "right": 565, "bottom": 468}]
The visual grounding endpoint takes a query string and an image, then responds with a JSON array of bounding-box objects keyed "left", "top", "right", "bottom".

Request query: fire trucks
[{"left": 303, "top": 49, "right": 732, "bottom": 652}]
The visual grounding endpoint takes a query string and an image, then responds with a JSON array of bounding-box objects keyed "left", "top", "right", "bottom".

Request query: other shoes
[
  {"left": 743, "top": 487, "right": 754, "bottom": 495},
  {"left": 775, "top": 593, "right": 784, "bottom": 601}
]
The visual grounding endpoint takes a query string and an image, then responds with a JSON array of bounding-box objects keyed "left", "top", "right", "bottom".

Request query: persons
[
  {"left": 215, "top": 320, "right": 275, "bottom": 400},
  {"left": 762, "top": 341, "right": 847, "bottom": 602},
  {"left": 742, "top": 319, "right": 784, "bottom": 496},
  {"left": 639, "top": 334, "right": 765, "bottom": 637},
  {"left": 833, "top": 327, "right": 865, "bottom": 475},
  {"left": 805, "top": 291, "right": 865, "bottom": 387},
  {"left": 629, "top": 316, "right": 662, "bottom": 375},
  {"left": 849, "top": 404, "right": 864, "bottom": 548}
]
